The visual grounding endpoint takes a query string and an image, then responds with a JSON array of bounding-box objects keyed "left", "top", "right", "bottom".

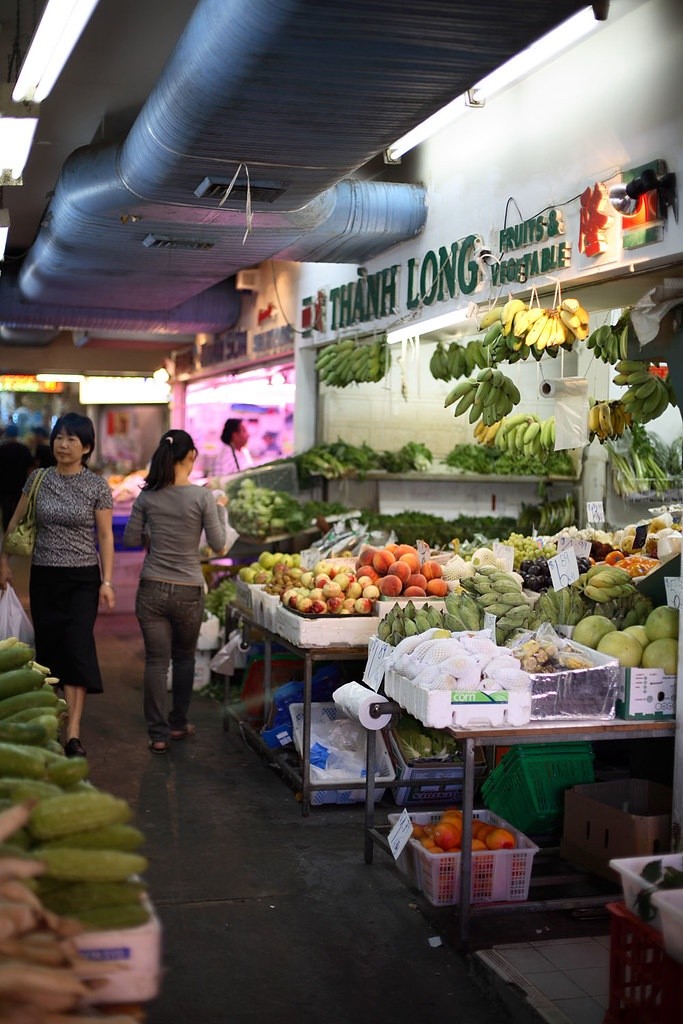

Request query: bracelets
[{"left": 102, "top": 581, "right": 114, "bottom": 587}]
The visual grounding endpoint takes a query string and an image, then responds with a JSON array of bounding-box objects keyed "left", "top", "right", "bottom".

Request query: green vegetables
[
  {"left": 226, "top": 478, "right": 517, "bottom": 533},
  {"left": 441, "top": 443, "right": 574, "bottom": 475},
  {"left": 391, "top": 714, "right": 459, "bottom": 761},
  {"left": 301, "top": 436, "right": 432, "bottom": 483},
  {"left": 602, "top": 424, "right": 683, "bottom": 496}
]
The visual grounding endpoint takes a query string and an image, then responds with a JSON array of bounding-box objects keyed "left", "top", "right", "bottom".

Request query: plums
[{"left": 517, "top": 557, "right": 591, "bottom": 590}]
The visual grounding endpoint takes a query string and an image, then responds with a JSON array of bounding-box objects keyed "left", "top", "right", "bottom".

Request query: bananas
[
  {"left": 315, "top": 340, "right": 393, "bottom": 388},
  {"left": 430, "top": 298, "right": 669, "bottom": 459},
  {"left": 378, "top": 566, "right": 650, "bottom": 647}
]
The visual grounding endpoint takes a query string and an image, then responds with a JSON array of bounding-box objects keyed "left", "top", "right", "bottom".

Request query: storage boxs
[
  {"left": 67, "top": 887, "right": 163, "bottom": 1006},
  {"left": 261, "top": 663, "right": 352, "bottom": 751},
  {"left": 387, "top": 720, "right": 488, "bottom": 805},
  {"left": 384, "top": 667, "right": 532, "bottom": 729},
  {"left": 234, "top": 575, "right": 448, "bottom": 648},
  {"left": 287, "top": 701, "right": 396, "bottom": 806},
  {"left": 558, "top": 779, "right": 673, "bottom": 885},
  {"left": 616, "top": 665, "right": 677, "bottom": 722},
  {"left": 111, "top": 515, "right": 145, "bottom": 553},
  {"left": 609, "top": 854, "right": 683, "bottom": 930},
  {"left": 530, "top": 638, "right": 620, "bottom": 722},
  {"left": 196, "top": 609, "right": 220, "bottom": 651},
  {"left": 387, "top": 808, "right": 541, "bottom": 905},
  {"left": 167, "top": 651, "right": 211, "bottom": 693},
  {"left": 605, "top": 900, "right": 683, "bottom": 1024}
]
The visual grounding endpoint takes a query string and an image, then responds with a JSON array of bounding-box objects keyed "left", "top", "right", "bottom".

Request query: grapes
[{"left": 464, "top": 533, "right": 558, "bottom": 570}]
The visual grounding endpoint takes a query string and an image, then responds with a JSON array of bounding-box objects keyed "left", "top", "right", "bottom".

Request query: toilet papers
[{"left": 332, "top": 681, "right": 392, "bottom": 730}]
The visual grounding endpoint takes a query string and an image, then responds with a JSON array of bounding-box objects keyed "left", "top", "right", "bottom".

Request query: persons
[
  {"left": 123, "top": 430, "right": 228, "bottom": 755},
  {"left": 0, "top": 420, "right": 57, "bottom": 531},
  {"left": 0, "top": 411, "right": 117, "bottom": 759},
  {"left": 220, "top": 419, "right": 253, "bottom": 472}
]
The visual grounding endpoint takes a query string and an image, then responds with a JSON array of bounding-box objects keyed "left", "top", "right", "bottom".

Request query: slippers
[
  {"left": 170, "top": 723, "right": 195, "bottom": 739},
  {"left": 150, "top": 742, "right": 165, "bottom": 754}
]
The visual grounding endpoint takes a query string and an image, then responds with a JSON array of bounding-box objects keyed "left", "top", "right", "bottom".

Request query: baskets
[
  {"left": 482, "top": 736, "right": 594, "bottom": 835},
  {"left": 389, "top": 809, "right": 540, "bottom": 905},
  {"left": 289, "top": 703, "right": 395, "bottom": 805}
]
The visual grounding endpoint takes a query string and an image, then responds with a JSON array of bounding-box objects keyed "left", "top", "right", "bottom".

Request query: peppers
[{"left": 604, "top": 551, "right": 658, "bottom": 577}]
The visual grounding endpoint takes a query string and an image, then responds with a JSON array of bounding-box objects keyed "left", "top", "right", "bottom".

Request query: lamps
[
  {"left": 607, "top": 169, "right": 659, "bottom": 218},
  {"left": 383, "top": 89, "right": 485, "bottom": 165},
  {"left": 387, "top": 300, "right": 479, "bottom": 345},
  {"left": 467, "top": 0, "right": 610, "bottom": 105}
]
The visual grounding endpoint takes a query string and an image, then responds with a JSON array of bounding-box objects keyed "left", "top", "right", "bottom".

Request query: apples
[
  {"left": 408, "top": 811, "right": 524, "bottom": 899},
  {"left": 621, "top": 520, "right": 683, "bottom": 554},
  {"left": 240, "top": 551, "right": 301, "bottom": 584},
  {"left": 281, "top": 562, "right": 380, "bottom": 615}
]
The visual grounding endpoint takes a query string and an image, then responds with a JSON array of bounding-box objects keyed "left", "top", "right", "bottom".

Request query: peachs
[{"left": 355, "top": 545, "right": 448, "bottom": 597}]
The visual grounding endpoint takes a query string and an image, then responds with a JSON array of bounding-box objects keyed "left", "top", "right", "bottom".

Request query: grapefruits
[{"left": 573, "top": 606, "right": 678, "bottom": 675}]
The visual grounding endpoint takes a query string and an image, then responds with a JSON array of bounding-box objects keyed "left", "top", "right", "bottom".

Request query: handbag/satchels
[
  {"left": 0, "top": 582, "right": 35, "bottom": 647},
  {"left": 5, "top": 466, "right": 50, "bottom": 556}
]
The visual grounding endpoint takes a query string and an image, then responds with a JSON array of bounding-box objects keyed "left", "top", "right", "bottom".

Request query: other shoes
[{"left": 66, "top": 737, "right": 87, "bottom": 758}]
